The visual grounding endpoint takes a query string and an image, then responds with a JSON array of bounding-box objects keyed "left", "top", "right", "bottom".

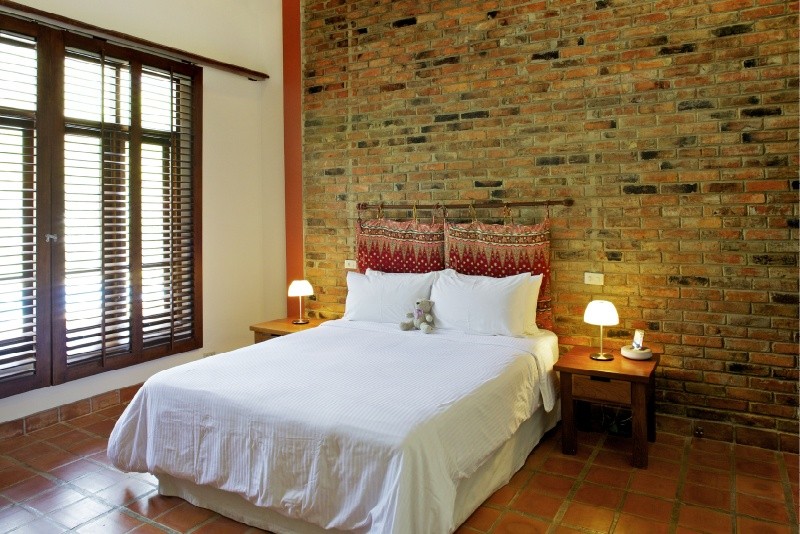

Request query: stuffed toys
[{"left": 399, "top": 299, "right": 434, "bottom": 333}]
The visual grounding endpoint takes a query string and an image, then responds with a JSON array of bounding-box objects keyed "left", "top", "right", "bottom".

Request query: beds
[{"left": 107, "top": 200, "right": 560, "bottom": 534}]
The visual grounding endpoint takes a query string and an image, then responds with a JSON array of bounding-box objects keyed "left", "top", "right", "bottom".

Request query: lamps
[
  {"left": 288, "top": 279, "right": 314, "bottom": 324},
  {"left": 583, "top": 300, "right": 619, "bottom": 360}
]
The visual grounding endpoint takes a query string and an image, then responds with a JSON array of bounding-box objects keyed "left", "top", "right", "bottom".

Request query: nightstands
[{"left": 249, "top": 318, "right": 330, "bottom": 343}]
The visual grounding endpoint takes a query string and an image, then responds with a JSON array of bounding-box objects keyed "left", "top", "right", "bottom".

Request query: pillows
[{"left": 343, "top": 267, "right": 545, "bottom": 338}]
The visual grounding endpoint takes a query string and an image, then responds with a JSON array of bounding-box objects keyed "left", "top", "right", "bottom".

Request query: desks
[{"left": 553, "top": 345, "right": 660, "bottom": 469}]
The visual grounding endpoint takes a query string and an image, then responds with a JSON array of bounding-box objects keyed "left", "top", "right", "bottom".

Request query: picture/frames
[{"left": 632, "top": 329, "right": 645, "bottom": 350}]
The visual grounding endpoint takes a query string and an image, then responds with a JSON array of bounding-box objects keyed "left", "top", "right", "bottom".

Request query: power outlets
[
  {"left": 345, "top": 260, "right": 357, "bottom": 269},
  {"left": 584, "top": 272, "right": 604, "bottom": 285}
]
